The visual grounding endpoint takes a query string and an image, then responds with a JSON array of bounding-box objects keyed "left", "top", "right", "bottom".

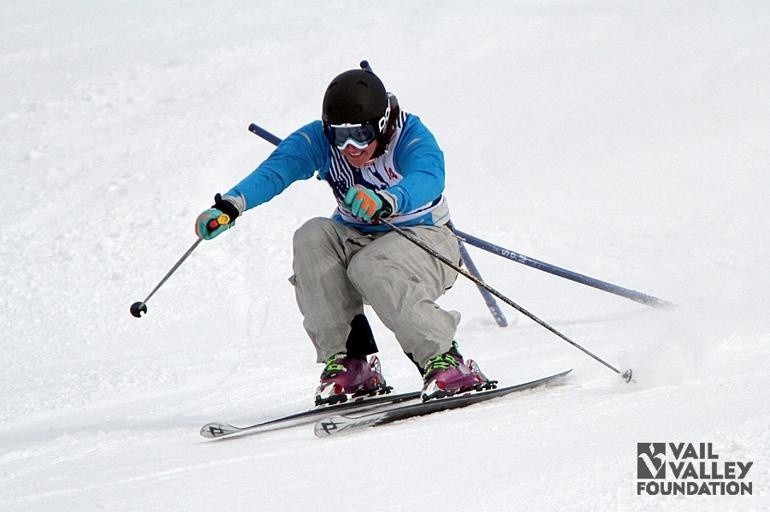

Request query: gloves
[
  {"left": 343, "top": 183, "right": 390, "bottom": 223},
  {"left": 194, "top": 199, "right": 240, "bottom": 240}
]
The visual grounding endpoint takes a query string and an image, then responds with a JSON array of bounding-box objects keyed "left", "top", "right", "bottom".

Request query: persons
[{"left": 195, "top": 70, "right": 488, "bottom": 401}]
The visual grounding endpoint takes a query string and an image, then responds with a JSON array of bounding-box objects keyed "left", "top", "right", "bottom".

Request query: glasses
[{"left": 323, "top": 122, "right": 376, "bottom": 150}]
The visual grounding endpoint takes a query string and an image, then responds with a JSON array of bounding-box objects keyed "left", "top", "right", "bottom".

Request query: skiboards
[{"left": 201, "top": 368, "right": 574, "bottom": 439}]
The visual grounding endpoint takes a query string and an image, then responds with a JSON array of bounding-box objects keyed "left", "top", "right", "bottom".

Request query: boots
[
  {"left": 420, "top": 344, "right": 498, "bottom": 403},
  {"left": 314, "top": 354, "right": 395, "bottom": 406}
]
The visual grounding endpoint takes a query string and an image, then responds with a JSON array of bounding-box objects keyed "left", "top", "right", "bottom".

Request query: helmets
[{"left": 321, "top": 68, "right": 393, "bottom": 168}]
